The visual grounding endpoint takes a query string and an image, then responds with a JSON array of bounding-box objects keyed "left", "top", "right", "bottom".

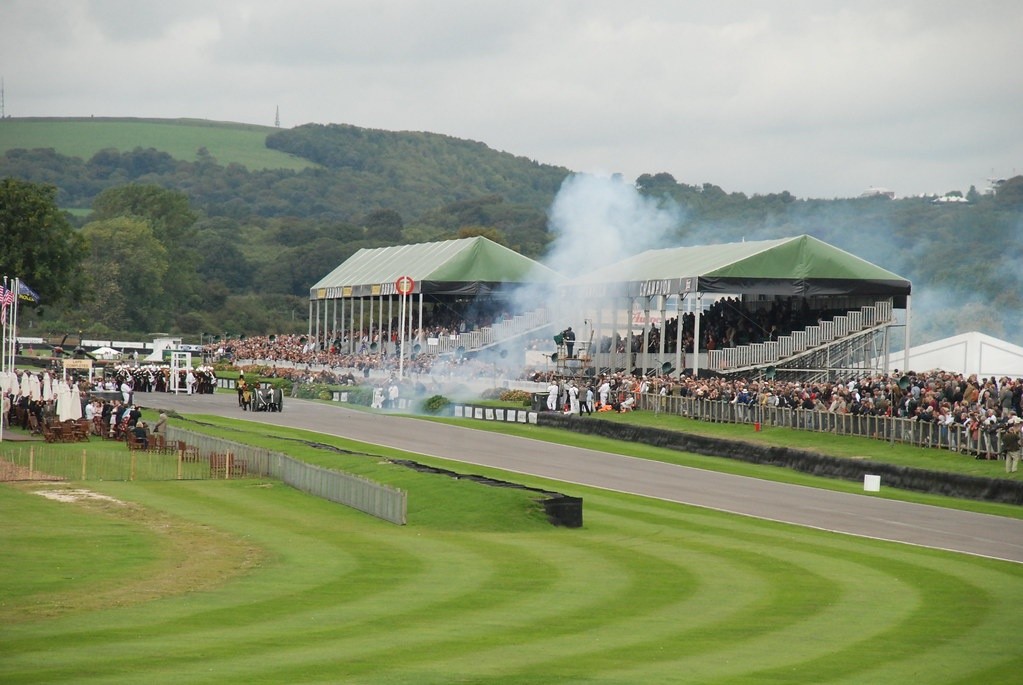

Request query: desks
[
  {"left": 62, "top": 423, "right": 82, "bottom": 439},
  {"left": 51, "top": 426, "right": 76, "bottom": 440}
]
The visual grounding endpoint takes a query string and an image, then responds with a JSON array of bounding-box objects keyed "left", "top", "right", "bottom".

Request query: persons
[
  {"left": 565, "top": 327, "right": 575, "bottom": 359},
  {"left": 0, "top": 297, "right": 1023, "bottom": 473},
  {"left": 236, "top": 370, "right": 247, "bottom": 407}
]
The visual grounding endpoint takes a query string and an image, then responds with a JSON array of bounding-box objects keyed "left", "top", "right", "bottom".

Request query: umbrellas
[
  {"left": 29, "top": 374, "right": 34, "bottom": 392},
  {"left": 22, "top": 373, "right": 30, "bottom": 396},
  {"left": 56, "top": 384, "right": 73, "bottom": 422},
  {"left": 51, "top": 379, "right": 59, "bottom": 397},
  {"left": 1, "top": 372, "right": 8, "bottom": 393},
  {"left": 42, "top": 374, "right": 52, "bottom": 401},
  {"left": 71, "top": 384, "right": 82, "bottom": 420},
  {"left": 9, "top": 373, "right": 19, "bottom": 396},
  {"left": 32, "top": 376, "right": 40, "bottom": 401}
]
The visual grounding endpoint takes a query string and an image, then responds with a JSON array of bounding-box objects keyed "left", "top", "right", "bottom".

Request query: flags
[{"left": 0, "top": 282, "right": 40, "bottom": 328}]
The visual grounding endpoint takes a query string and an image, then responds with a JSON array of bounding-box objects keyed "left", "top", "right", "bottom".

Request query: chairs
[{"left": 26, "top": 414, "right": 249, "bottom": 477}]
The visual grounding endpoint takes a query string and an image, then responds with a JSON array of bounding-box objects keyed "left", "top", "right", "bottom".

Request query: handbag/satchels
[{"left": 576, "top": 394, "right": 578, "bottom": 399}]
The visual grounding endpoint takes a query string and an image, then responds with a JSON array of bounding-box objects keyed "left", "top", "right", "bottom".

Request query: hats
[
  {"left": 240, "top": 370, "right": 244, "bottom": 378},
  {"left": 115, "top": 363, "right": 214, "bottom": 371},
  {"left": 742, "top": 389, "right": 748, "bottom": 394}
]
